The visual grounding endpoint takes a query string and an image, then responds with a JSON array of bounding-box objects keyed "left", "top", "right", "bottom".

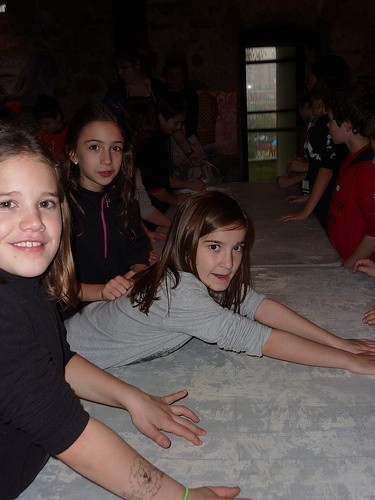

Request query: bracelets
[{"left": 181, "top": 484, "right": 191, "bottom": 500}]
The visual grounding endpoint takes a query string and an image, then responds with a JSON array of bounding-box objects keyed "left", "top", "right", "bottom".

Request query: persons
[
  {"left": 58, "top": 109, "right": 151, "bottom": 300},
  {"left": 1, "top": 45, "right": 374, "bottom": 269},
  {"left": 0, "top": 124, "right": 255, "bottom": 500},
  {"left": 353, "top": 258, "right": 375, "bottom": 326},
  {"left": 61, "top": 189, "right": 375, "bottom": 376}
]
